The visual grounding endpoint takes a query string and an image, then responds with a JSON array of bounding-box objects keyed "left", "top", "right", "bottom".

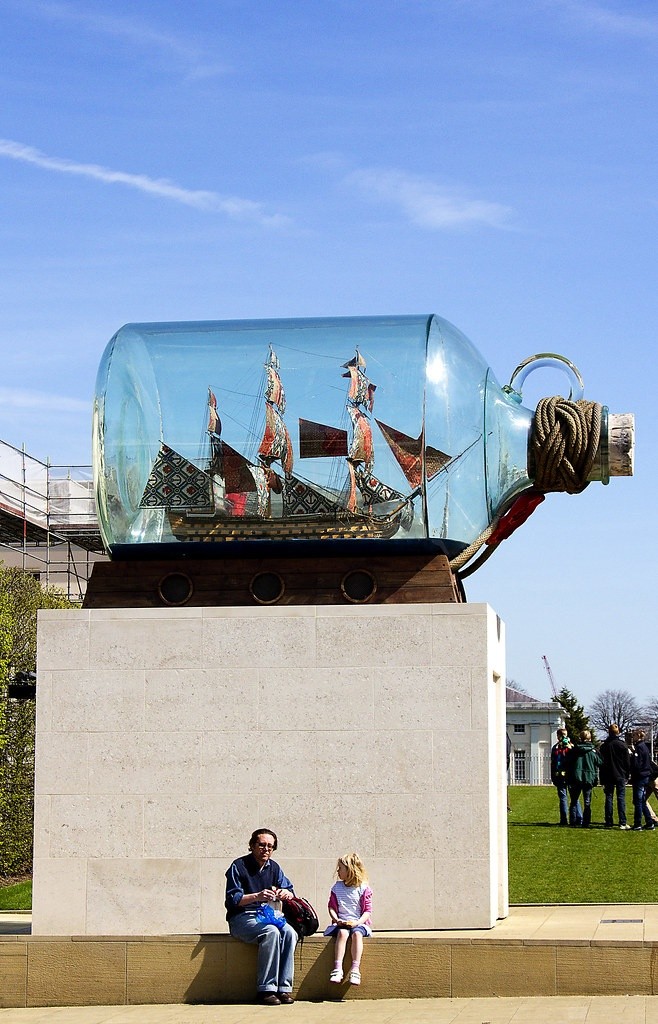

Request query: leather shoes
[
  {"left": 275, "top": 990, "right": 294, "bottom": 1003},
  {"left": 260, "top": 991, "right": 281, "bottom": 1005}
]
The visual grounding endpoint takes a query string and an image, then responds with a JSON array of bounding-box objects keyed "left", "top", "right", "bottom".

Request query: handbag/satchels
[{"left": 282, "top": 898, "right": 319, "bottom": 940}]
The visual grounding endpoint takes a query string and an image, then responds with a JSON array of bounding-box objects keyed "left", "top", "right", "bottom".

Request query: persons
[
  {"left": 550, "top": 723, "right": 658, "bottom": 830},
  {"left": 328, "top": 852, "right": 374, "bottom": 985},
  {"left": 224, "top": 828, "right": 301, "bottom": 1006}
]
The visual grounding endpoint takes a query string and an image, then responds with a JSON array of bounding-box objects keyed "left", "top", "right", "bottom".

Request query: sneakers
[
  {"left": 328, "top": 969, "right": 344, "bottom": 983},
  {"left": 349, "top": 969, "right": 361, "bottom": 985}
]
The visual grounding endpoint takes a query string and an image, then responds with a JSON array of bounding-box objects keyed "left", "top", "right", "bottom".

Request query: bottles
[{"left": 93, "top": 312, "right": 634, "bottom": 569}]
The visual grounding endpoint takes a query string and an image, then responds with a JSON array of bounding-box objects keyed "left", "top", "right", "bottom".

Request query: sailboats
[{"left": 138, "top": 342, "right": 493, "bottom": 542}]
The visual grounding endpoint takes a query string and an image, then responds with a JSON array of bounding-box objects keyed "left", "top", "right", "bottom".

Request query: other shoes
[
  {"left": 627, "top": 825, "right": 642, "bottom": 831},
  {"left": 641, "top": 824, "right": 655, "bottom": 831},
  {"left": 559, "top": 821, "right": 591, "bottom": 828},
  {"left": 604, "top": 823, "right": 613, "bottom": 828},
  {"left": 619, "top": 823, "right": 632, "bottom": 829}
]
